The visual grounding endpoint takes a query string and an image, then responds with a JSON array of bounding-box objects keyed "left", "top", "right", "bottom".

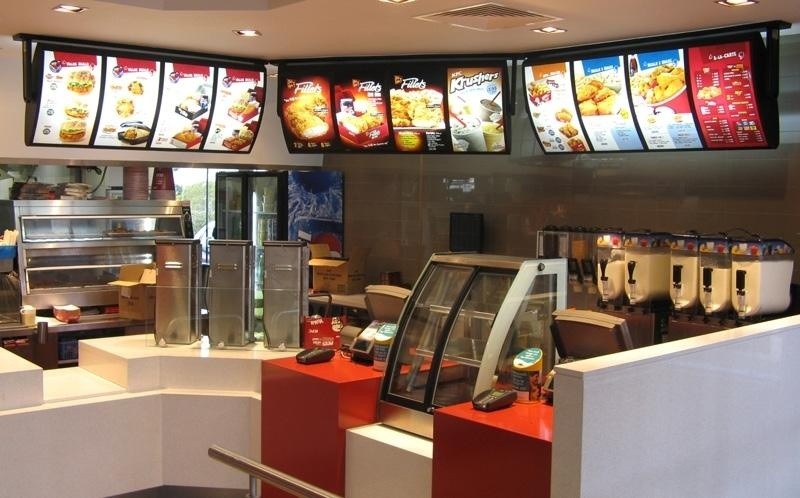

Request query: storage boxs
[
  {"left": 105, "top": 264, "right": 157, "bottom": 322},
  {"left": 307, "top": 243, "right": 371, "bottom": 295}
]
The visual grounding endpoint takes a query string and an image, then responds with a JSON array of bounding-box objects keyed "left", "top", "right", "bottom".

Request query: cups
[
  {"left": 480, "top": 98, "right": 502, "bottom": 121},
  {"left": 19, "top": 307, "right": 36, "bottom": 326},
  {"left": 452, "top": 125, "right": 488, "bottom": 152},
  {"left": 482, "top": 122, "right": 504, "bottom": 151}
]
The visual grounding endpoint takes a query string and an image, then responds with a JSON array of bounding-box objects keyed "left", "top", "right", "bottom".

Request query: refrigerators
[{"left": 213, "top": 167, "right": 346, "bottom": 339}]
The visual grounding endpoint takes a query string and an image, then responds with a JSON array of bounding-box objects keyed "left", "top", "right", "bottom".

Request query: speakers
[{"left": 447, "top": 211, "right": 485, "bottom": 254}]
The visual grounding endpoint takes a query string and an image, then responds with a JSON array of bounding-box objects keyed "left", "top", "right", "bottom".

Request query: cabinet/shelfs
[
  {"left": 0, "top": 198, "right": 192, "bottom": 313},
  {"left": 377, "top": 250, "right": 569, "bottom": 439}
]
